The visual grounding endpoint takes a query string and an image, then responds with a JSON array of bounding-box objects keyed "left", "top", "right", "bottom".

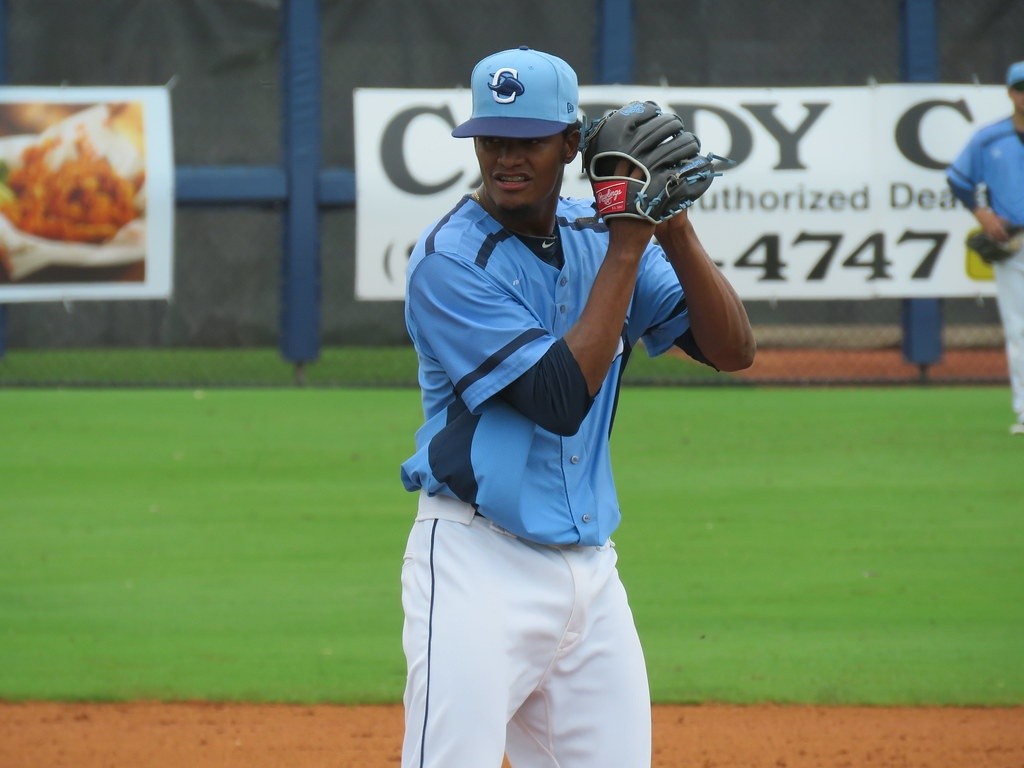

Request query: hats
[
  {"left": 451, "top": 45, "right": 580, "bottom": 137},
  {"left": 1008, "top": 61, "right": 1024, "bottom": 87}
]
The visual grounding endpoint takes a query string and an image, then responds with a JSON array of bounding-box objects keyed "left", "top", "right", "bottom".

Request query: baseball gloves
[{"left": 577, "top": 97, "right": 741, "bottom": 226}]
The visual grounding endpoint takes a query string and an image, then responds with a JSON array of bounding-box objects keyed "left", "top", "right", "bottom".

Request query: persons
[
  {"left": 400, "top": 48, "right": 757, "bottom": 768},
  {"left": 946, "top": 61, "right": 1024, "bottom": 435}
]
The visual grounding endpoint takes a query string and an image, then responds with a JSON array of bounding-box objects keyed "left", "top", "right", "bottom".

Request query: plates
[{"left": 0, "top": 133, "right": 147, "bottom": 267}]
